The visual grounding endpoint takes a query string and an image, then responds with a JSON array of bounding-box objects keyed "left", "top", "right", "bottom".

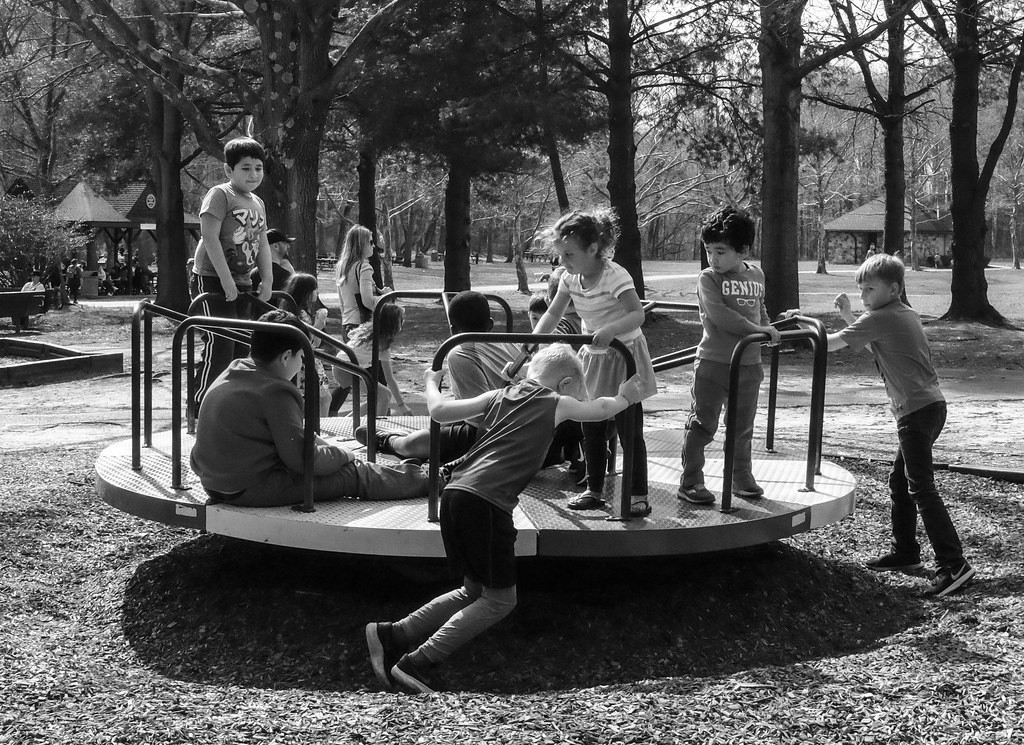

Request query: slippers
[
  {"left": 630, "top": 501, "right": 652, "bottom": 516},
  {"left": 567, "top": 493, "right": 605, "bottom": 508}
]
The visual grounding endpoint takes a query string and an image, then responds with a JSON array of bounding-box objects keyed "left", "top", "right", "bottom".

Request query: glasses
[{"left": 370, "top": 240, "right": 374, "bottom": 245}]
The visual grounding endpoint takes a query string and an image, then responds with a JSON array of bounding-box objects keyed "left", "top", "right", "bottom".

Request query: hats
[{"left": 266, "top": 228, "right": 297, "bottom": 244}]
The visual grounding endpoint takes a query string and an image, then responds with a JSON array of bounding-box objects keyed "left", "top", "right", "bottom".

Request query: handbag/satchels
[{"left": 354, "top": 292, "right": 380, "bottom": 324}]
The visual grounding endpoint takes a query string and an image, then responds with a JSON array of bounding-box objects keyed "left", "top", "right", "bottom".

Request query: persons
[
  {"left": 501, "top": 206, "right": 658, "bottom": 517},
  {"left": 192, "top": 136, "right": 276, "bottom": 423},
  {"left": 785, "top": 252, "right": 976, "bottom": 598},
  {"left": 831, "top": 231, "right": 956, "bottom": 270},
  {"left": 278, "top": 273, "right": 333, "bottom": 419},
  {"left": 335, "top": 223, "right": 398, "bottom": 414},
  {"left": 250, "top": 226, "right": 295, "bottom": 304},
  {"left": 673, "top": 206, "right": 782, "bottom": 506},
  {"left": 186, "top": 309, "right": 449, "bottom": 509},
  {"left": 525, "top": 294, "right": 549, "bottom": 332},
  {"left": 542, "top": 266, "right": 611, "bottom": 484},
  {"left": 13, "top": 246, "right": 200, "bottom": 325},
  {"left": 328, "top": 304, "right": 416, "bottom": 416},
  {"left": 363, "top": 344, "right": 640, "bottom": 690},
  {"left": 355, "top": 289, "right": 526, "bottom": 466}
]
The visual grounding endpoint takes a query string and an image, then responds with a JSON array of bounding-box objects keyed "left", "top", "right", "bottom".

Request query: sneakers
[
  {"left": 365, "top": 622, "right": 395, "bottom": 691},
  {"left": 676, "top": 483, "right": 715, "bottom": 504},
  {"left": 865, "top": 549, "right": 924, "bottom": 571},
  {"left": 732, "top": 482, "right": 764, "bottom": 498},
  {"left": 925, "top": 559, "right": 976, "bottom": 597},
  {"left": 391, "top": 654, "right": 451, "bottom": 694},
  {"left": 354, "top": 424, "right": 408, "bottom": 453}
]
